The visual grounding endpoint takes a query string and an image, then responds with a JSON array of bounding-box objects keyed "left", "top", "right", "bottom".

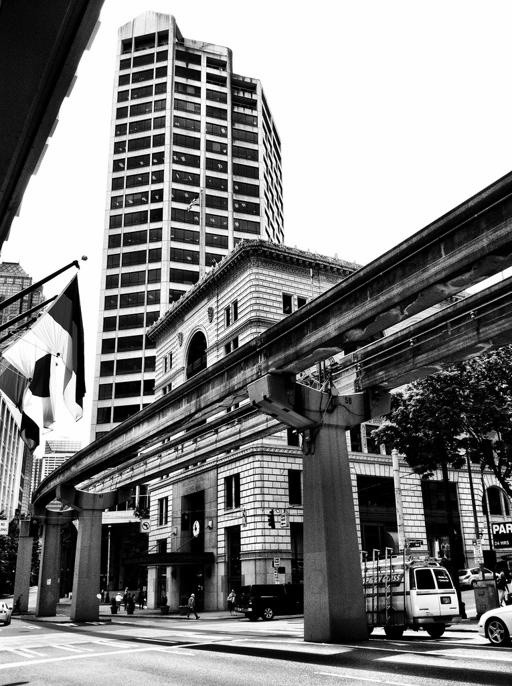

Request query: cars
[
  {"left": 457, "top": 565, "right": 508, "bottom": 588},
  {"left": 0, "top": 601, "right": 13, "bottom": 626},
  {"left": 476, "top": 602, "right": 512, "bottom": 647}
]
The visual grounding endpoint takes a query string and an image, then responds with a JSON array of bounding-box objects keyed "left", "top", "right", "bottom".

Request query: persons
[
  {"left": 186, "top": 593, "right": 201, "bottom": 620},
  {"left": 494, "top": 571, "right": 510, "bottom": 608},
  {"left": 98, "top": 585, "right": 146, "bottom": 615},
  {"left": 226, "top": 589, "right": 237, "bottom": 617}
]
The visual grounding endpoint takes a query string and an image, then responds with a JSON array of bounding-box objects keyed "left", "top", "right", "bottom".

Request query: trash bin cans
[{"left": 473, "top": 580, "right": 501, "bottom": 619}]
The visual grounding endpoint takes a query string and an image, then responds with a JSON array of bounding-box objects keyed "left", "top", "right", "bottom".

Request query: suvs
[{"left": 233, "top": 582, "right": 303, "bottom": 621}]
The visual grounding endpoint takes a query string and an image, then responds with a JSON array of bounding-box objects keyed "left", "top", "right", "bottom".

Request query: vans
[{"left": 360, "top": 554, "right": 461, "bottom": 640}]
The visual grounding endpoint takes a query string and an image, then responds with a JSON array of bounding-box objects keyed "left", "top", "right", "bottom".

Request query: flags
[{"left": 0, "top": 273, "right": 87, "bottom": 462}]
